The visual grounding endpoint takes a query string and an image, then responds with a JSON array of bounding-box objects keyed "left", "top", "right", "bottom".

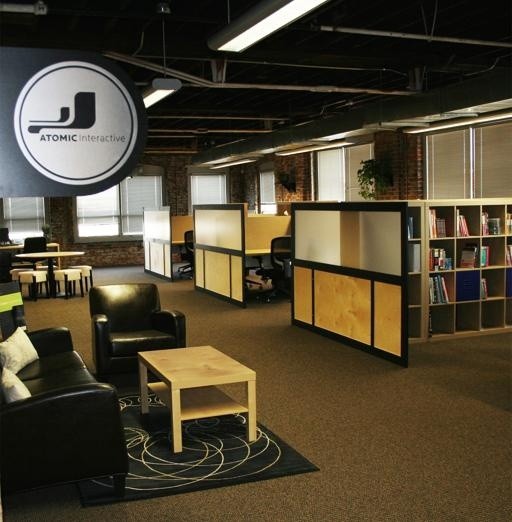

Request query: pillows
[{"left": 1, "top": 326, "right": 39, "bottom": 404}]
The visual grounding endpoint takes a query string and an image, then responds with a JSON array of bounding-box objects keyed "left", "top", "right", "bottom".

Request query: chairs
[
  {"left": 178, "top": 229, "right": 194, "bottom": 279},
  {"left": 89, "top": 284, "right": 187, "bottom": 382},
  {"left": 0, "top": 228, "right": 17, "bottom": 247},
  {"left": 25, "top": 239, "right": 48, "bottom": 269},
  {"left": 251, "top": 236, "right": 291, "bottom": 304},
  {"left": 33, "top": 242, "right": 60, "bottom": 269}
]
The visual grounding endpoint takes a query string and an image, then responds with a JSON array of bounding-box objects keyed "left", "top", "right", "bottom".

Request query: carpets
[{"left": 74, "top": 373, "right": 319, "bottom": 508}]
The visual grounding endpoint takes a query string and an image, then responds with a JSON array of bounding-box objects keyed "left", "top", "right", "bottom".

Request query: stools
[{"left": 10, "top": 264, "right": 93, "bottom": 302}]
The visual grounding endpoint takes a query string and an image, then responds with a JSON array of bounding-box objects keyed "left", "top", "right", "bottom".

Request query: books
[{"left": 429, "top": 207, "right": 512, "bottom": 304}]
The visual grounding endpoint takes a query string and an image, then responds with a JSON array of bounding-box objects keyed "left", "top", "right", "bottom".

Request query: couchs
[{"left": 1, "top": 326, "right": 129, "bottom": 497}]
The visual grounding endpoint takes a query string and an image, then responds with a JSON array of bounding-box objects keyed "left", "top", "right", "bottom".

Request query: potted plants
[
  {"left": 277, "top": 167, "right": 297, "bottom": 193},
  {"left": 357, "top": 157, "right": 394, "bottom": 200}
]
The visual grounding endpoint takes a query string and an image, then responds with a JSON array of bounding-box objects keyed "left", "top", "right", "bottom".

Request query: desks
[
  {"left": 15, "top": 251, "right": 84, "bottom": 298},
  {"left": 138, "top": 344, "right": 257, "bottom": 453},
  {"left": 1, "top": 246, "right": 25, "bottom": 253}
]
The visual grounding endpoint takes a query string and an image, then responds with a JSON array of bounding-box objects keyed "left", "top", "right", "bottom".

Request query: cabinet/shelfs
[{"left": 408, "top": 197, "right": 512, "bottom": 346}]
